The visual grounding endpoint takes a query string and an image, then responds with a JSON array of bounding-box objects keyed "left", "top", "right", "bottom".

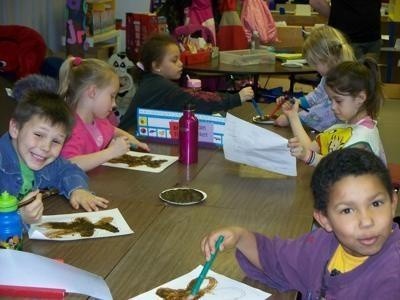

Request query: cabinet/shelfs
[{"left": 64, "top": 0, "right": 121, "bottom": 62}]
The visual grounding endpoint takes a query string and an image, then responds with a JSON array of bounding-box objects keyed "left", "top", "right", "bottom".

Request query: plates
[
  {"left": 158, "top": 188, "right": 208, "bottom": 206},
  {"left": 253, "top": 115, "right": 275, "bottom": 124},
  {"left": 281, "top": 62, "right": 303, "bottom": 68}
]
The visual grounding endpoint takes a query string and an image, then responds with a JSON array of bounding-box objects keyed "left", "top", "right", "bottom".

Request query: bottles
[
  {"left": 187, "top": 78, "right": 201, "bottom": 91},
  {"left": 256, "top": 32, "right": 260, "bottom": 49},
  {"left": 0, "top": 190, "right": 24, "bottom": 251},
  {"left": 179, "top": 102, "right": 198, "bottom": 165},
  {"left": 249, "top": 34, "right": 256, "bottom": 49}
]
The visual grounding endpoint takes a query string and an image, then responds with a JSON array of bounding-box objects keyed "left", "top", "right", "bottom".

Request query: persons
[
  {"left": 276, "top": 24, "right": 356, "bottom": 133},
  {"left": 281, "top": 57, "right": 388, "bottom": 170},
  {"left": 56, "top": 55, "right": 149, "bottom": 173},
  {"left": 116, "top": 34, "right": 254, "bottom": 136},
  {"left": 200, "top": 148, "right": 400, "bottom": 300},
  {"left": 0, "top": 91, "right": 109, "bottom": 223},
  {"left": 309, "top": 0, "right": 383, "bottom": 59}
]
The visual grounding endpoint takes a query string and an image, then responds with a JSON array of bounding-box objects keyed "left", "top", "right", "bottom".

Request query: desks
[{"left": 183, "top": 48, "right": 319, "bottom": 102}]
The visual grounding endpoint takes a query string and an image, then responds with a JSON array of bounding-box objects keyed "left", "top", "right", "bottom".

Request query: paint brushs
[
  {"left": 250, "top": 97, "right": 265, "bottom": 121},
  {"left": 115, "top": 136, "right": 144, "bottom": 152},
  {"left": 285, "top": 95, "right": 310, "bottom": 115},
  {"left": 270, "top": 96, "right": 290, "bottom": 117},
  {"left": 17, "top": 186, "right": 60, "bottom": 208},
  {"left": 191, "top": 236, "right": 222, "bottom": 292}
]
